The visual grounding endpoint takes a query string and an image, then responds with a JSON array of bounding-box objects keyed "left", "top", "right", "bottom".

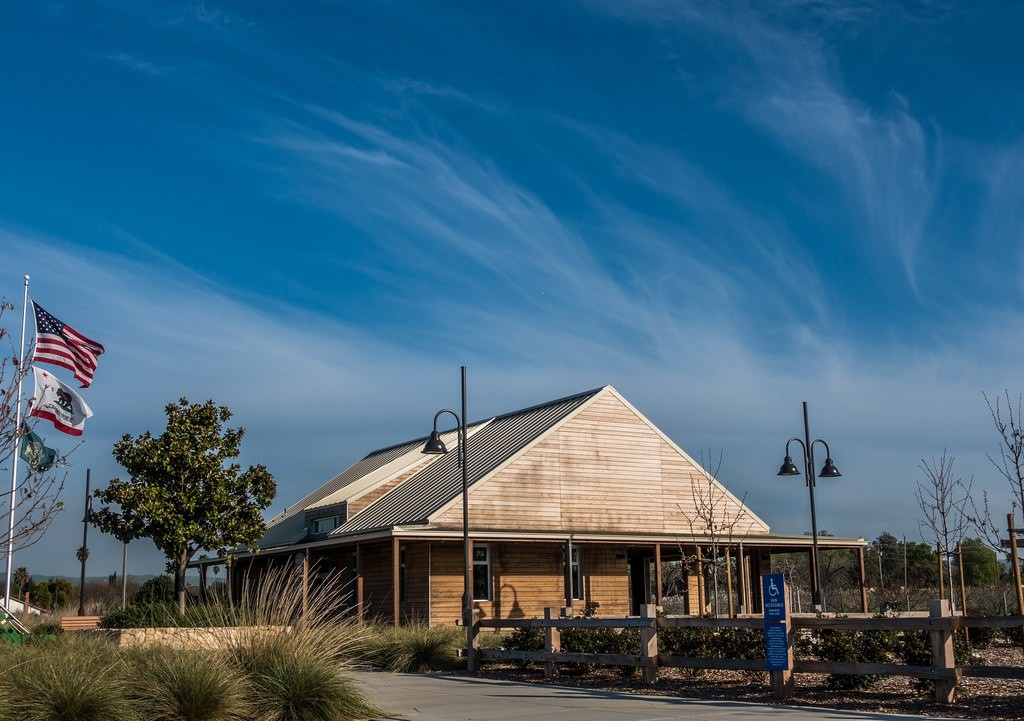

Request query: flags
[
  {"left": 28, "top": 298, "right": 106, "bottom": 388},
  {"left": 27, "top": 365, "right": 93, "bottom": 436},
  {"left": 20, "top": 421, "right": 57, "bottom": 473}
]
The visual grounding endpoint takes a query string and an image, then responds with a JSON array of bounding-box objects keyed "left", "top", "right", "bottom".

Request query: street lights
[
  {"left": 774, "top": 399, "right": 842, "bottom": 615},
  {"left": 77, "top": 468, "right": 96, "bottom": 616},
  {"left": 421, "top": 364, "right": 473, "bottom": 626}
]
